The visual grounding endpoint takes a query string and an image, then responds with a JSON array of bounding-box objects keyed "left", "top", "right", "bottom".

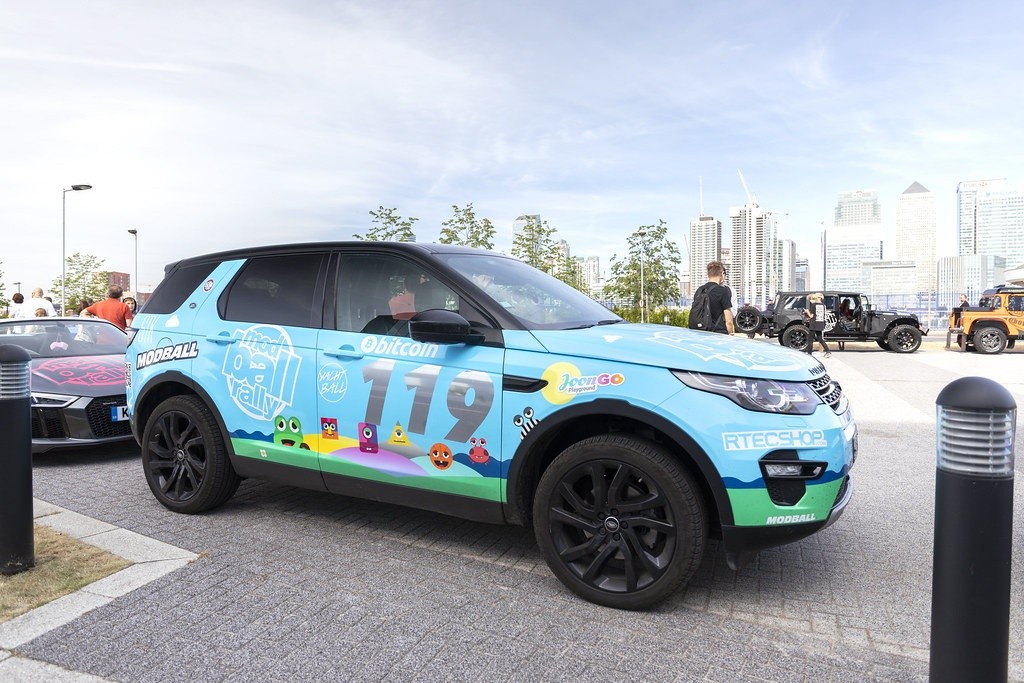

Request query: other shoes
[{"left": 824, "top": 353, "right": 831, "bottom": 358}]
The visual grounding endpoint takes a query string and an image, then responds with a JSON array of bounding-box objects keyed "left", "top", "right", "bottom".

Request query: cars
[{"left": 0, "top": 317, "right": 135, "bottom": 454}]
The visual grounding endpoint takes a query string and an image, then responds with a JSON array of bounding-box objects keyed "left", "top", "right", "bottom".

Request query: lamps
[
  {"left": 0, "top": 344, "right": 32, "bottom": 399},
  {"left": 934, "top": 377, "right": 1017, "bottom": 479}
]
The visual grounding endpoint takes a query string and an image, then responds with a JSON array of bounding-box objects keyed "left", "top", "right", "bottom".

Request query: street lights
[
  {"left": 13, "top": 282, "right": 21, "bottom": 296},
  {"left": 60, "top": 184, "right": 94, "bottom": 315},
  {"left": 638, "top": 231, "right": 647, "bottom": 323},
  {"left": 127, "top": 229, "right": 139, "bottom": 315}
]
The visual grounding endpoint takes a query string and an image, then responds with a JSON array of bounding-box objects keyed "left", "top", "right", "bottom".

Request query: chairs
[{"left": 39, "top": 332, "right": 89, "bottom": 354}]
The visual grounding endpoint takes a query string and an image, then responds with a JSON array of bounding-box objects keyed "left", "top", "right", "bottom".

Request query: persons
[
  {"left": 390, "top": 272, "right": 425, "bottom": 319},
  {"left": 6, "top": 284, "right": 138, "bottom": 343},
  {"left": 694, "top": 261, "right": 739, "bottom": 336},
  {"left": 957, "top": 294, "right": 970, "bottom": 307},
  {"left": 802, "top": 293, "right": 855, "bottom": 358}
]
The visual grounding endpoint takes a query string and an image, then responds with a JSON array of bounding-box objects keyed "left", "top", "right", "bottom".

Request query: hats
[{"left": 816, "top": 293, "right": 824, "bottom": 300}]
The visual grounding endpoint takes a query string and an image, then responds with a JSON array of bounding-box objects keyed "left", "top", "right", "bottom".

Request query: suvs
[
  {"left": 124, "top": 232, "right": 863, "bottom": 611},
  {"left": 948, "top": 286, "right": 1024, "bottom": 355},
  {"left": 768, "top": 289, "right": 931, "bottom": 354}
]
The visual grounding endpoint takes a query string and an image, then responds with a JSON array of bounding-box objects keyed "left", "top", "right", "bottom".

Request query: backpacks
[{"left": 688, "top": 284, "right": 722, "bottom": 332}]
